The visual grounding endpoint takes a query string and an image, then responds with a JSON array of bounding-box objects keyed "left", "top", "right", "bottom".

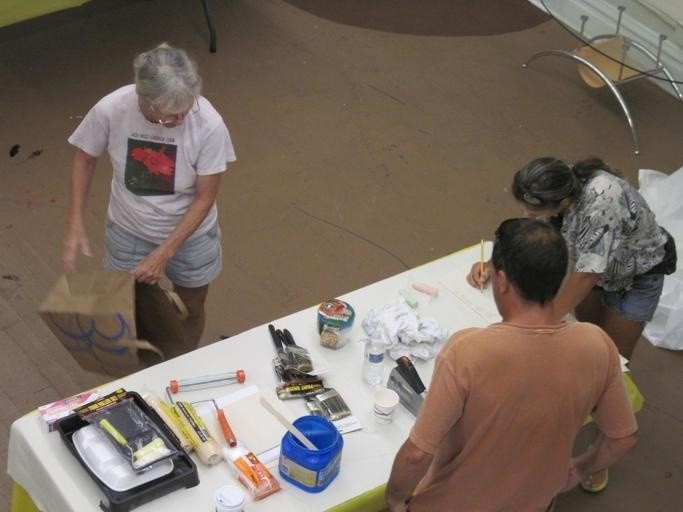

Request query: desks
[
  {"left": 518, "top": 2, "right": 683, "bottom": 159},
  {"left": 6, "top": 237, "right": 648, "bottom": 511}
]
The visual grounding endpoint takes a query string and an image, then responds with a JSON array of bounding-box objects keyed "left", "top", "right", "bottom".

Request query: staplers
[{"left": 386, "top": 356, "right": 425, "bottom": 417}]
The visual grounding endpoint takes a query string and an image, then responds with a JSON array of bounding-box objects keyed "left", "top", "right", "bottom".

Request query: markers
[
  {"left": 398, "top": 288, "right": 417, "bottom": 309},
  {"left": 413, "top": 282, "right": 438, "bottom": 297}
]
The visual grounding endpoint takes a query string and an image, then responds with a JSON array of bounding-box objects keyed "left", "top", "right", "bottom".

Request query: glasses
[{"left": 146, "top": 95, "right": 200, "bottom": 128}]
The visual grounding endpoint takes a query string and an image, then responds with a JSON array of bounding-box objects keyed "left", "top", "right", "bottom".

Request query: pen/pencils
[{"left": 481, "top": 238, "right": 484, "bottom": 293}]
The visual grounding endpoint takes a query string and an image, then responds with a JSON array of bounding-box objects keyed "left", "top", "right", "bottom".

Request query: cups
[
  {"left": 212, "top": 484, "right": 246, "bottom": 512},
  {"left": 373, "top": 388, "right": 399, "bottom": 425}
]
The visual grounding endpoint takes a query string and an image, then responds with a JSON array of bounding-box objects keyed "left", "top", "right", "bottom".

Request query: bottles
[
  {"left": 361, "top": 329, "right": 386, "bottom": 388},
  {"left": 275, "top": 414, "right": 344, "bottom": 492}
]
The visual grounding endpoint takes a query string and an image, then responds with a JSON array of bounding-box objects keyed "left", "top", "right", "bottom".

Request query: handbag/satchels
[
  {"left": 659, "top": 224, "right": 676, "bottom": 275},
  {"left": 37, "top": 270, "right": 189, "bottom": 377}
]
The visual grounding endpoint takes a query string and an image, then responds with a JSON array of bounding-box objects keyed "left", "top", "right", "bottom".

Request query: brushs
[
  {"left": 142, "top": 392, "right": 236, "bottom": 465},
  {"left": 99, "top": 418, "right": 178, "bottom": 473}
]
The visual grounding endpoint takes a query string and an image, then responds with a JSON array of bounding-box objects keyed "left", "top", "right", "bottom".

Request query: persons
[
  {"left": 64, "top": 44, "right": 238, "bottom": 367},
  {"left": 465, "top": 156, "right": 665, "bottom": 494},
  {"left": 384, "top": 216, "right": 640, "bottom": 512}
]
